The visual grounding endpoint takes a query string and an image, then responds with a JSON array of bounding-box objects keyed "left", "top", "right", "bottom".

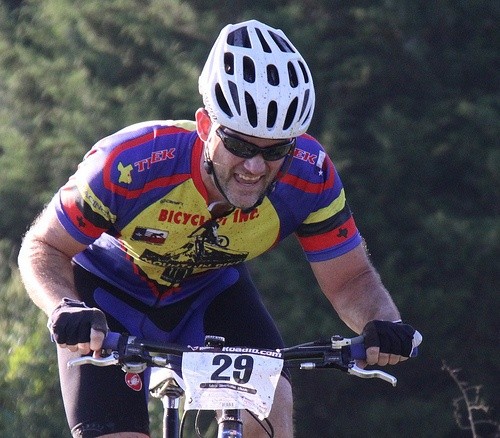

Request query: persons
[{"left": 17, "top": 19, "right": 415, "bottom": 438}]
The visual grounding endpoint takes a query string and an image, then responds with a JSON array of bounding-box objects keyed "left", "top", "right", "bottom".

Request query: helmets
[{"left": 198, "top": 20, "right": 315, "bottom": 140}]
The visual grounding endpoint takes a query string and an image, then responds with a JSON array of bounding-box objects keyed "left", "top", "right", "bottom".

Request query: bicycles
[{"left": 49, "top": 329, "right": 418, "bottom": 438}]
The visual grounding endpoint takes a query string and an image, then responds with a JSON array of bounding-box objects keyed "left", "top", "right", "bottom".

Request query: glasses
[{"left": 216, "top": 129, "right": 295, "bottom": 161}]
